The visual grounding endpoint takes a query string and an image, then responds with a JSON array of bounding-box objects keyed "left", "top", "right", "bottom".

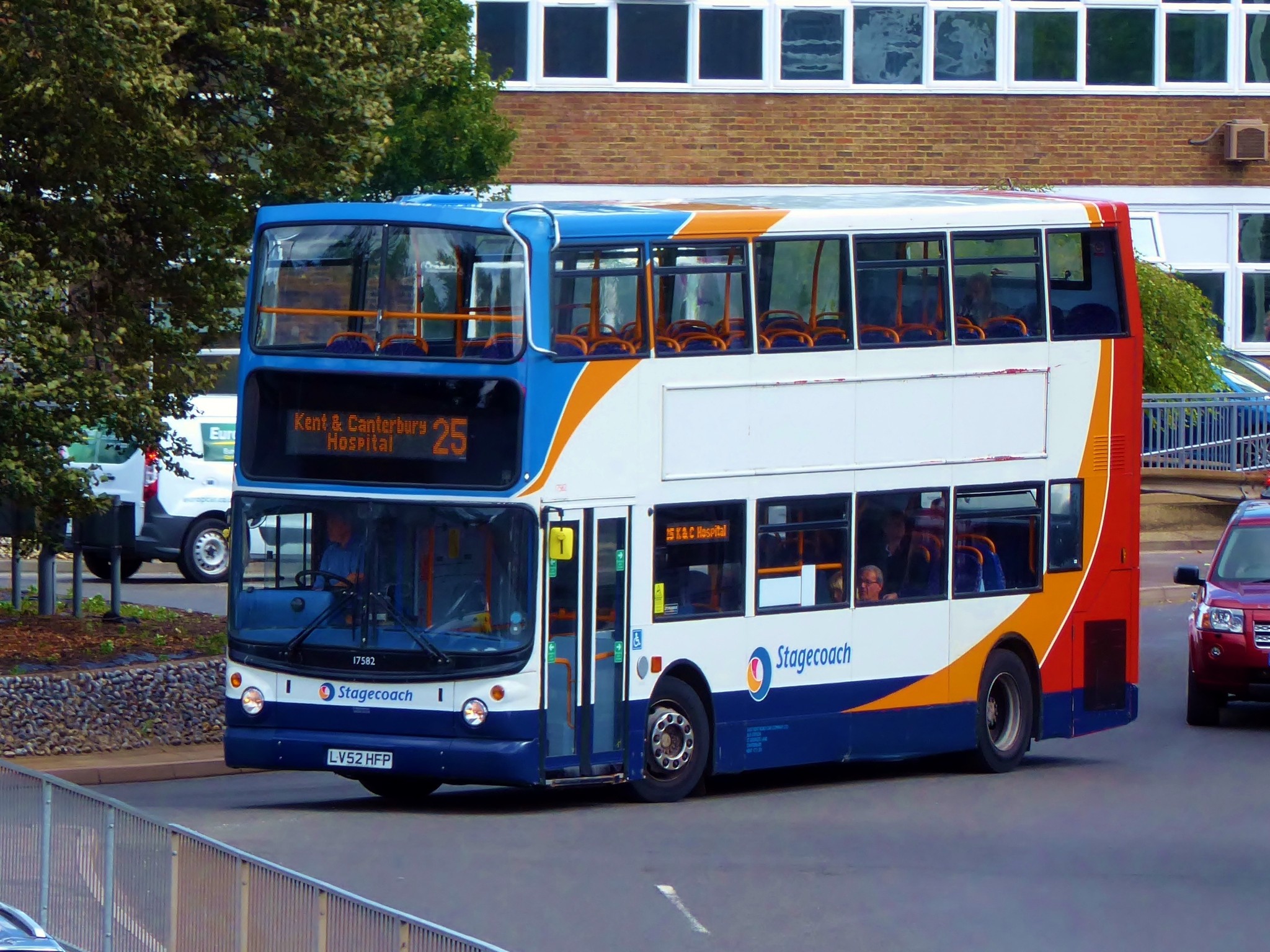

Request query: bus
[{"left": 222, "top": 182, "right": 1144, "bottom": 801}]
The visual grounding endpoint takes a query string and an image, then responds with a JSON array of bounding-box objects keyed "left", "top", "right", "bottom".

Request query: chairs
[
  {"left": 910, "top": 530, "right": 945, "bottom": 594},
  {"left": 905, "top": 544, "right": 931, "bottom": 596},
  {"left": 956, "top": 533, "right": 1007, "bottom": 589},
  {"left": 323, "top": 332, "right": 376, "bottom": 354},
  {"left": 775, "top": 538, "right": 818, "bottom": 566},
  {"left": 938, "top": 546, "right": 985, "bottom": 592},
  {"left": 379, "top": 334, "right": 432, "bottom": 357},
  {"left": 480, "top": 302, "right": 1117, "bottom": 358}
]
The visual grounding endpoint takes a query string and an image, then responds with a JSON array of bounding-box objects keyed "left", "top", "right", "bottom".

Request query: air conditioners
[{"left": 1223, "top": 120, "right": 1269, "bottom": 162}]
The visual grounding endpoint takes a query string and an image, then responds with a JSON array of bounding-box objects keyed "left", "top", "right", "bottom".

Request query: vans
[{"left": 25, "top": 347, "right": 239, "bottom": 582}]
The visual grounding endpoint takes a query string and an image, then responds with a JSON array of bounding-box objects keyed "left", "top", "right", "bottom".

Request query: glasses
[{"left": 859, "top": 579, "right": 878, "bottom": 587}]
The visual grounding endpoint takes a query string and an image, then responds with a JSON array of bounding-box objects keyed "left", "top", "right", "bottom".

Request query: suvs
[{"left": 1172, "top": 500, "right": 1269, "bottom": 726}]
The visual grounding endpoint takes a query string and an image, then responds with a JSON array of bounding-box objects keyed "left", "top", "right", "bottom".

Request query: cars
[{"left": 1145, "top": 343, "right": 1270, "bottom": 467}]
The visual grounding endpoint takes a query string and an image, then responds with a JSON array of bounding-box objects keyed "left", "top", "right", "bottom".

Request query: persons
[
  {"left": 845, "top": 501, "right": 924, "bottom": 604},
  {"left": 958, "top": 273, "right": 1010, "bottom": 327},
  {"left": 309, "top": 508, "right": 370, "bottom": 625}
]
